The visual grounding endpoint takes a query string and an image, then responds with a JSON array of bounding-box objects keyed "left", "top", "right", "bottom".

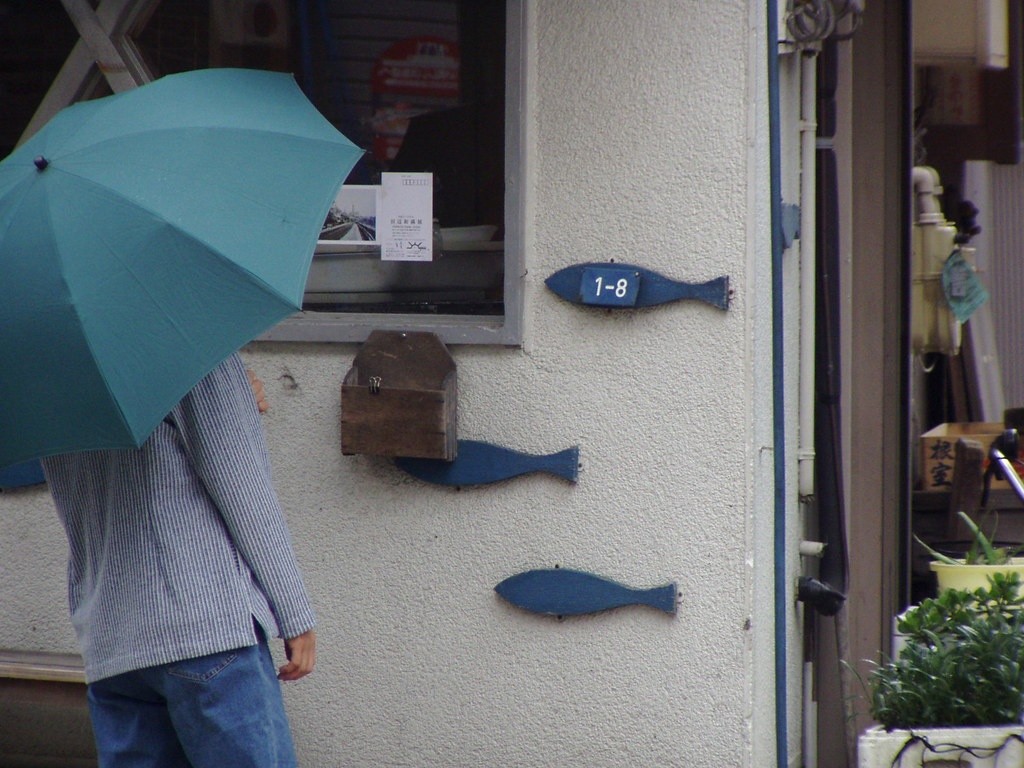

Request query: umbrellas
[{"left": 0, "top": 68, "right": 367, "bottom": 470}]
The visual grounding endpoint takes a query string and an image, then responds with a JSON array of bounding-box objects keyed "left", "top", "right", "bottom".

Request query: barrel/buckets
[{"left": 929, "top": 557, "right": 1024, "bottom": 610}]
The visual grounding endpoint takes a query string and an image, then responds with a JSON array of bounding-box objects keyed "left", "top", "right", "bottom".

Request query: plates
[{"left": 440, "top": 225, "right": 497, "bottom": 240}]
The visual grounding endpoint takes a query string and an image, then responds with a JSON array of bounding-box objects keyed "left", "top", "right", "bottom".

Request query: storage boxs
[{"left": 918, "top": 420, "right": 1015, "bottom": 493}]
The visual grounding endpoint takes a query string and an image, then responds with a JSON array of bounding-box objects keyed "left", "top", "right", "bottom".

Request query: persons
[{"left": 41, "top": 350, "right": 321, "bottom": 768}]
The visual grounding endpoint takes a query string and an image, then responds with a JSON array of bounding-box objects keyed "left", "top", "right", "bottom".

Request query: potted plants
[
  {"left": 911, "top": 500, "right": 1024, "bottom": 610},
  {"left": 837, "top": 568, "right": 1024, "bottom": 767}
]
